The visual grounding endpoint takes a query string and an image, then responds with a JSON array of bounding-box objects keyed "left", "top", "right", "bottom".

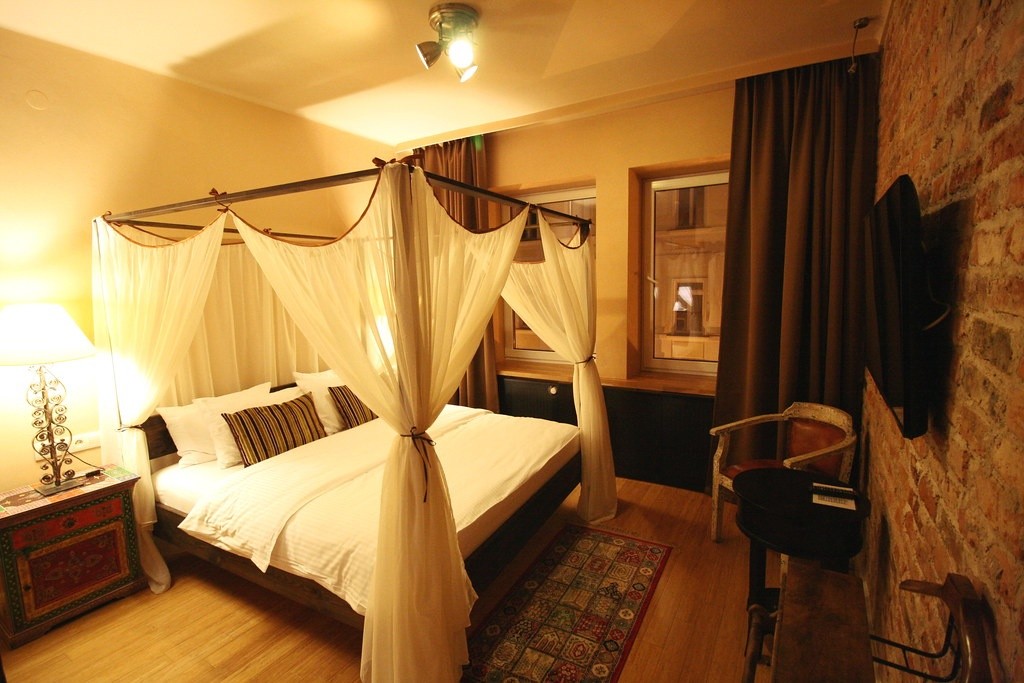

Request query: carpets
[{"left": 462, "top": 522, "right": 674, "bottom": 683}]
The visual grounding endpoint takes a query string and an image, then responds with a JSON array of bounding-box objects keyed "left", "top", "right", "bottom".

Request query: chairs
[{"left": 709, "top": 401, "right": 858, "bottom": 582}]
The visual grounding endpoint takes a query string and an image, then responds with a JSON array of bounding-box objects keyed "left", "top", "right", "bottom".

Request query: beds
[{"left": 129, "top": 384, "right": 582, "bottom": 631}]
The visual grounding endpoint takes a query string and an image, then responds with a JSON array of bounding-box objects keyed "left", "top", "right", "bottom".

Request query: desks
[{"left": 732, "top": 468, "right": 873, "bottom": 635}]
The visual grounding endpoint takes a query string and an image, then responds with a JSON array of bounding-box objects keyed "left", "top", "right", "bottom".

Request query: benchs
[{"left": 769, "top": 556, "right": 875, "bottom": 683}]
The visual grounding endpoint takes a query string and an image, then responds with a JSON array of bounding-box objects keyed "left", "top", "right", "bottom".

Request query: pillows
[
  {"left": 328, "top": 385, "right": 379, "bottom": 429},
  {"left": 194, "top": 381, "right": 272, "bottom": 469},
  {"left": 221, "top": 391, "right": 328, "bottom": 468},
  {"left": 293, "top": 369, "right": 348, "bottom": 437},
  {"left": 156, "top": 404, "right": 216, "bottom": 467}
]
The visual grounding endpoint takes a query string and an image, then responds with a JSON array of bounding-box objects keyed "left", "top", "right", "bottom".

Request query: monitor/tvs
[{"left": 863, "top": 174, "right": 942, "bottom": 439}]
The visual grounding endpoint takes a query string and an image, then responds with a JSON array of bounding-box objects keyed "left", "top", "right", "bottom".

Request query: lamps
[
  {"left": 415, "top": 3, "right": 482, "bottom": 81},
  {"left": 0, "top": 303, "right": 100, "bottom": 494}
]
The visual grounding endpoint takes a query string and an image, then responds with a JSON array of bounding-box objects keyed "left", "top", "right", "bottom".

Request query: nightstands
[{"left": 0, "top": 463, "right": 148, "bottom": 648}]
